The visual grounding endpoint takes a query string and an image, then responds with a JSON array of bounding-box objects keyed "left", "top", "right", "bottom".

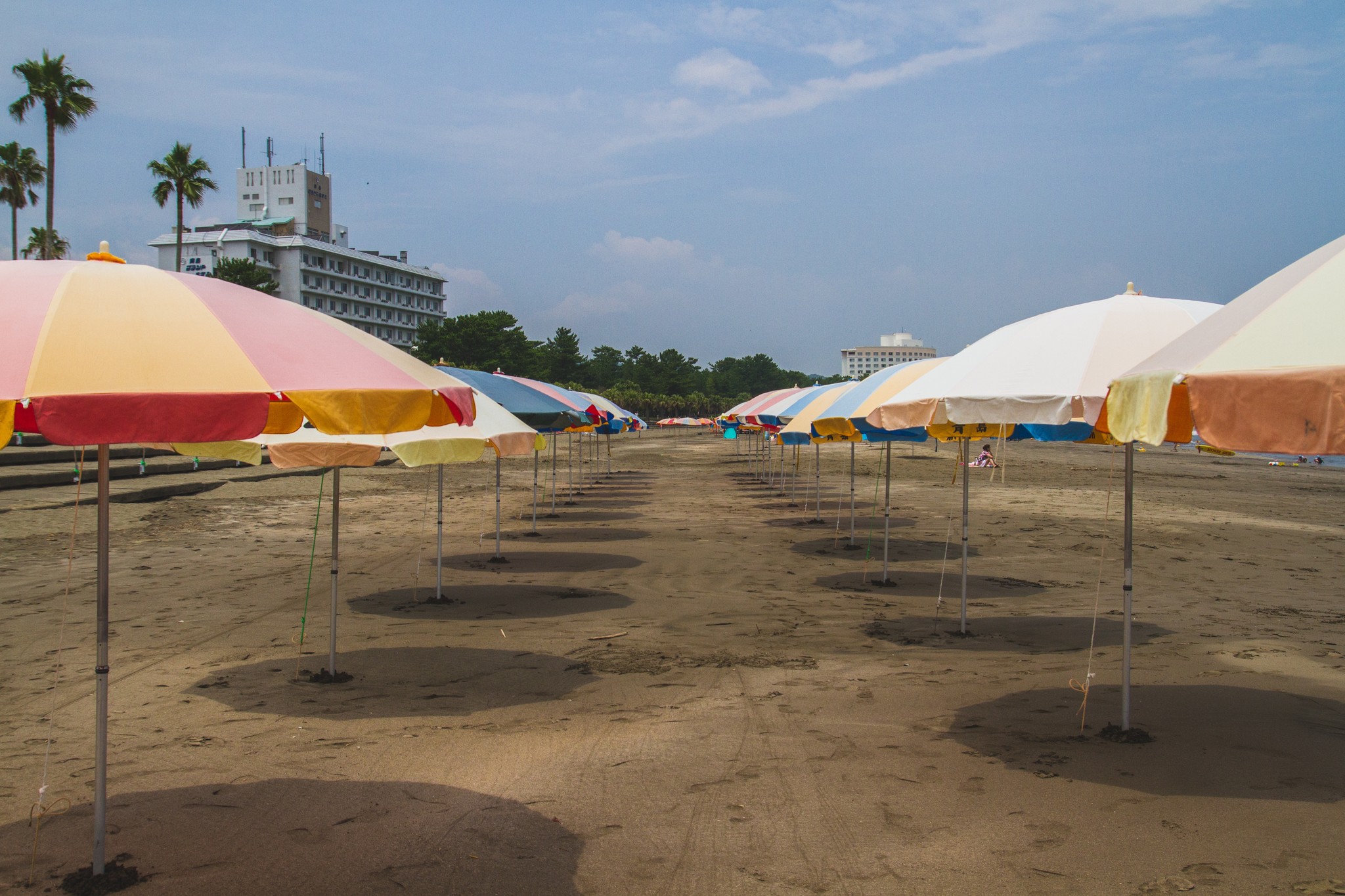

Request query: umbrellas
[
  {"left": 0, "top": 239, "right": 481, "bottom": 896},
  {"left": 116, "top": 393, "right": 547, "bottom": 686},
  {"left": 418, "top": 358, "right": 930, "bottom": 600},
  {"left": 867, "top": 281, "right": 1227, "bottom": 731},
  {"left": 1094, "top": 233, "right": 1344, "bottom": 465},
  {"left": 808, "top": 343, "right": 1140, "bottom": 636}
]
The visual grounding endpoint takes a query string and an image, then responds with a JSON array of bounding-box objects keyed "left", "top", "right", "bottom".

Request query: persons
[
  {"left": 1296, "top": 455, "right": 1324, "bottom": 464},
  {"left": 975, "top": 444, "right": 1001, "bottom": 468}
]
